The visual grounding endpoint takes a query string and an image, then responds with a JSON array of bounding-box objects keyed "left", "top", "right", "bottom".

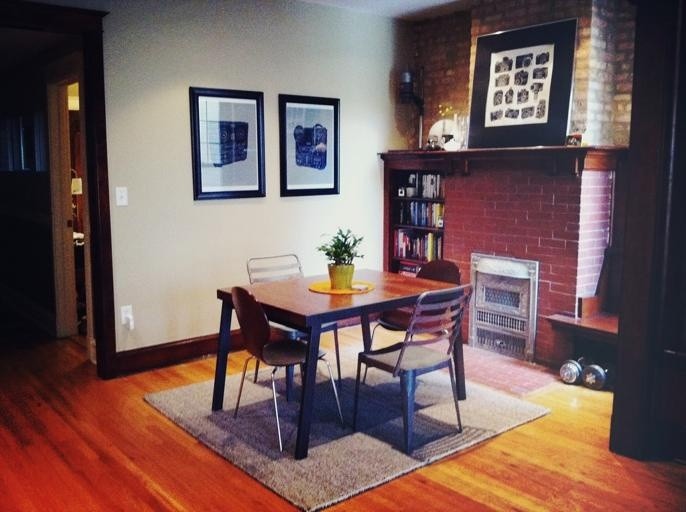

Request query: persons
[{"left": 568, "top": 137, "right": 579, "bottom": 145}]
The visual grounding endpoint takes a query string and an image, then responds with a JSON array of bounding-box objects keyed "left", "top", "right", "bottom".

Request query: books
[
  {"left": 398, "top": 260, "right": 423, "bottom": 278},
  {"left": 399, "top": 201, "right": 444, "bottom": 229},
  {"left": 2, "top": 111, "right": 48, "bottom": 172},
  {"left": 393, "top": 229, "right": 443, "bottom": 263},
  {"left": 421, "top": 174, "right": 440, "bottom": 198}
]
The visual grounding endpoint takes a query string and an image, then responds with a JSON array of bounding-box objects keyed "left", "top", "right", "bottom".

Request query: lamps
[
  {"left": 67, "top": 81, "right": 80, "bottom": 112},
  {"left": 71, "top": 169, "right": 83, "bottom": 196}
]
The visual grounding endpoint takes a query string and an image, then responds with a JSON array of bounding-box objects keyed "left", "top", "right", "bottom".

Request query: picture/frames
[
  {"left": 189, "top": 86, "right": 267, "bottom": 200},
  {"left": 278, "top": 94, "right": 341, "bottom": 197},
  {"left": 468, "top": 18, "right": 578, "bottom": 145}
]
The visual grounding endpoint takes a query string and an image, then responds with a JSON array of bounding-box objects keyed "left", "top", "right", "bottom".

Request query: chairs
[
  {"left": 229, "top": 286, "right": 345, "bottom": 453},
  {"left": 353, "top": 283, "right": 474, "bottom": 456},
  {"left": 246, "top": 253, "right": 341, "bottom": 401},
  {"left": 364, "top": 260, "right": 466, "bottom": 399}
]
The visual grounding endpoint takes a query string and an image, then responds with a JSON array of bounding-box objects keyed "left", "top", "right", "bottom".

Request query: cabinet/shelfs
[{"left": 379, "top": 152, "right": 444, "bottom": 329}]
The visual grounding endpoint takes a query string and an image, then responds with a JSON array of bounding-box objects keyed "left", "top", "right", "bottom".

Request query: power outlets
[{"left": 121, "top": 306, "right": 134, "bottom": 330}]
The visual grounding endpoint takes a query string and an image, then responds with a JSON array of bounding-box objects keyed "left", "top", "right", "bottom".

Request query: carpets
[{"left": 142, "top": 334, "right": 549, "bottom": 512}]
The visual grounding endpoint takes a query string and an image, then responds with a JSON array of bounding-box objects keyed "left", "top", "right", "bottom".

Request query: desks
[{"left": 211, "top": 269, "right": 466, "bottom": 460}]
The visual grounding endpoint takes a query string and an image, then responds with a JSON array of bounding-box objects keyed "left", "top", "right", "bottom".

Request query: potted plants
[{"left": 319, "top": 230, "right": 364, "bottom": 288}]
